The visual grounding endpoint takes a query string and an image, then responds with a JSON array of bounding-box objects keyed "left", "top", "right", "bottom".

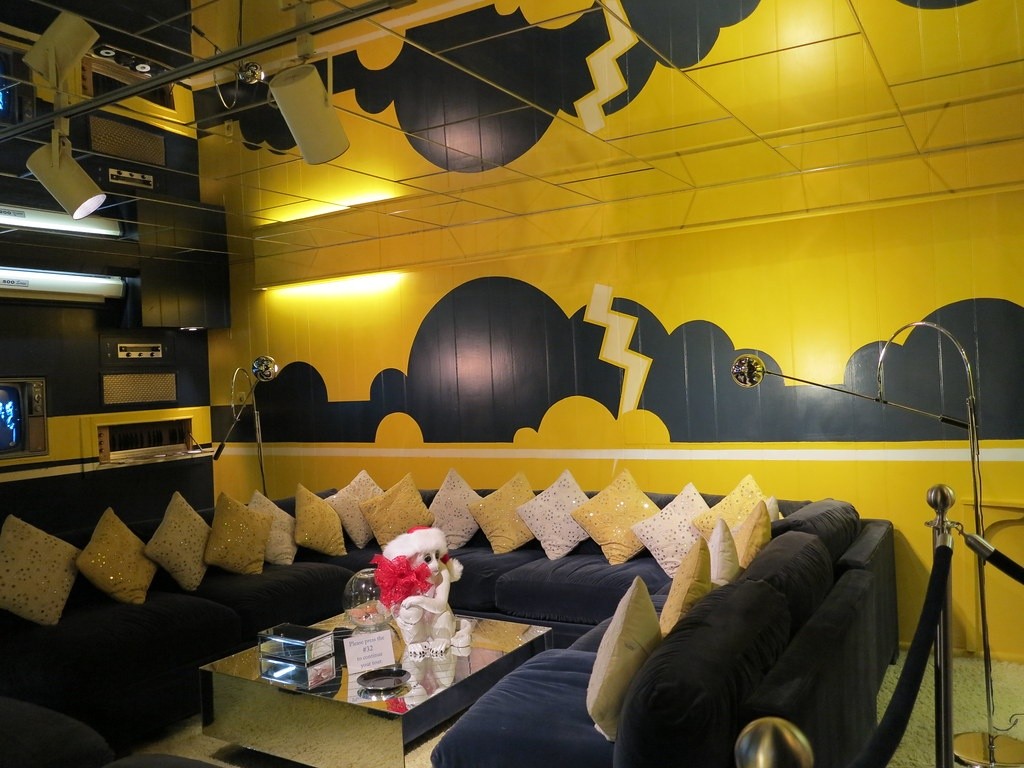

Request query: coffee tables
[{"left": 198, "top": 600, "right": 555, "bottom": 768}]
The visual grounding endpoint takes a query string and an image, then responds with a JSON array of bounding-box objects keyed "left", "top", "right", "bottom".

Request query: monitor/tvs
[{"left": 0, "top": 376, "right": 51, "bottom": 461}]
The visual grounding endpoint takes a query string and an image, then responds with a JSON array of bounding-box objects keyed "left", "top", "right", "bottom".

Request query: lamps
[
  {"left": 25, "top": 117, "right": 106, "bottom": 220},
  {"left": 269, "top": 33, "right": 350, "bottom": 166},
  {"left": 21, "top": 11, "right": 100, "bottom": 113}
]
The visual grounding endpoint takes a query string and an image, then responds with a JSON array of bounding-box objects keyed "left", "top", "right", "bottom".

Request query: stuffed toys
[{"left": 369, "top": 527, "right": 472, "bottom": 655}]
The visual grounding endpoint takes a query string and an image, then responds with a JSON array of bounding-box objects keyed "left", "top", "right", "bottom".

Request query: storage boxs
[
  {"left": 256, "top": 622, "right": 336, "bottom": 667},
  {"left": 258, "top": 657, "right": 337, "bottom": 690}
]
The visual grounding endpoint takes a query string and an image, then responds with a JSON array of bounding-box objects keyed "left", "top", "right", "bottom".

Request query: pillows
[
  {"left": 733, "top": 500, "right": 772, "bottom": 577},
  {"left": 660, "top": 536, "right": 712, "bottom": 638},
  {"left": 516, "top": 470, "right": 591, "bottom": 561},
  {"left": 707, "top": 517, "right": 740, "bottom": 591},
  {"left": 571, "top": 469, "right": 662, "bottom": 566},
  {"left": 204, "top": 491, "right": 273, "bottom": 575},
  {"left": 294, "top": 483, "right": 348, "bottom": 557},
  {"left": 359, "top": 471, "right": 435, "bottom": 553},
  {"left": 468, "top": 470, "right": 537, "bottom": 554},
  {"left": 247, "top": 489, "right": 298, "bottom": 566},
  {"left": 0, "top": 515, "right": 83, "bottom": 625},
  {"left": 584, "top": 575, "right": 664, "bottom": 742},
  {"left": 729, "top": 495, "right": 779, "bottom": 538},
  {"left": 691, "top": 473, "right": 784, "bottom": 543},
  {"left": 427, "top": 469, "right": 484, "bottom": 550},
  {"left": 142, "top": 492, "right": 212, "bottom": 592},
  {"left": 75, "top": 507, "right": 158, "bottom": 604},
  {"left": 630, "top": 483, "right": 711, "bottom": 580},
  {"left": 323, "top": 470, "right": 385, "bottom": 549}
]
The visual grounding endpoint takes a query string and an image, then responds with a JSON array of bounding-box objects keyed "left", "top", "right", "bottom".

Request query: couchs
[{"left": 0, "top": 469, "right": 899, "bottom": 768}]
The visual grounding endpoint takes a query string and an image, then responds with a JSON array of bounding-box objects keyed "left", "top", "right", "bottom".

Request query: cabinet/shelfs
[{"left": 128, "top": 261, "right": 231, "bottom": 333}]
